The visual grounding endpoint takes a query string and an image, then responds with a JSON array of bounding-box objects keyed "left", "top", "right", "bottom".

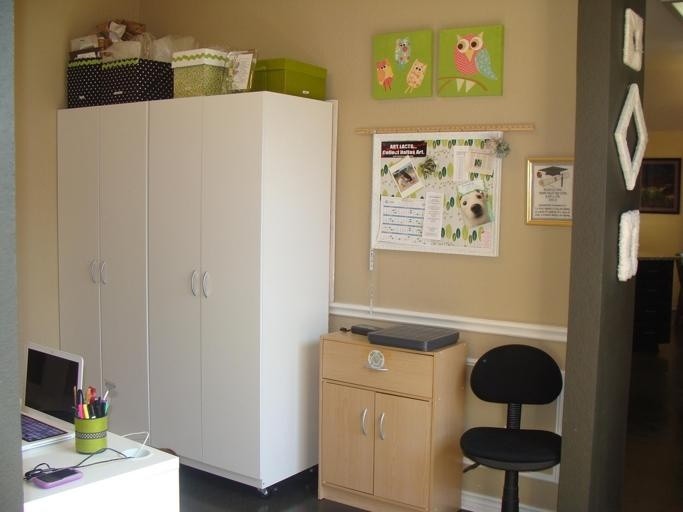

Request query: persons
[{"left": 398, "top": 168, "right": 416, "bottom": 185}]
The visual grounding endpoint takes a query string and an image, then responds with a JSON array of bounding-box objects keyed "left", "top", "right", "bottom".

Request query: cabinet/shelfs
[
  {"left": 317, "top": 332, "right": 466, "bottom": 511},
  {"left": 56, "top": 92, "right": 338, "bottom": 496}
]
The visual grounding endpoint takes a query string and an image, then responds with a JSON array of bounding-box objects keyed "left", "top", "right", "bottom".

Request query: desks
[{"left": 21, "top": 427, "right": 181, "bottom": 510}]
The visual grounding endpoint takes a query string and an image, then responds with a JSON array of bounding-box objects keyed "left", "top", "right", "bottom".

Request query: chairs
[{"left": 460, "top": 342, "right": 561, "bottom": 511}]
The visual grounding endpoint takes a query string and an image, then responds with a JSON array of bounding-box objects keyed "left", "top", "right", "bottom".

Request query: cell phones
[{"left": 33, "top": 466, "right": 83, "bottom": 488}]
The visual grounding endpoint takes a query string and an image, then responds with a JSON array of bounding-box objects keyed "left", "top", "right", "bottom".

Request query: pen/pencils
[{"left": 73, "top": 383, "right": 116, "bottom": 419}]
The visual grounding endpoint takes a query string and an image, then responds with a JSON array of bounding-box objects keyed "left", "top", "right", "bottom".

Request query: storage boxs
[{"left": 65, "top": 35, "right": 327, "bottom": 108}]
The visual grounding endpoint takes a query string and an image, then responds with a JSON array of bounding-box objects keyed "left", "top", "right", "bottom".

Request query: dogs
[{"left": 461, "top": 190, "right": 488, "bottom": 223}]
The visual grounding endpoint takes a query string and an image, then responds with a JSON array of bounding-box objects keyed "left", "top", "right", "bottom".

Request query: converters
[{"left": 351, "top": 323, "right": 383, "bottom": 334}]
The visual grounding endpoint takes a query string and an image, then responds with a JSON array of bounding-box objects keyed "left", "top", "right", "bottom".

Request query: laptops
[{"left": 19, "top": 342, "right": 84, "bottom": 453}]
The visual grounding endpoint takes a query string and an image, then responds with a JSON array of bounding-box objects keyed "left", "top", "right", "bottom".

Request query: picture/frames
[
  {"left": 640, "top": 157, "right": 682, "bottom": 215},
  {"left": 524, "top": 156, "right": 573, "bottom": 226}
]
